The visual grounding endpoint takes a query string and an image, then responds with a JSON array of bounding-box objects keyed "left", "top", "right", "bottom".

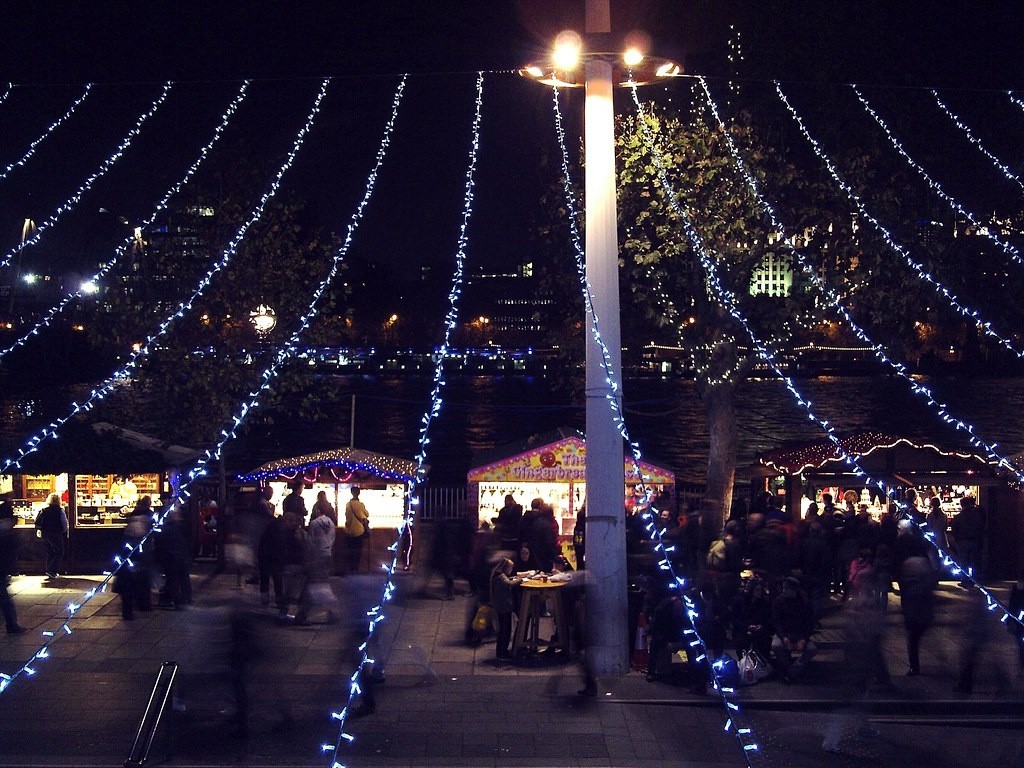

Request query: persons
[
  {"left": 248, "top": 481, "right": 370, "bottom": 624},
  {"left": 109, "top": 475, "right": 124, "bottom": 499},
  {"left": 0, "top": 492, "right": 27, "bottom": 635},
  {"left": 437, "top": 494, "right": 560, "bottom": 658},
  {"left": 198, "top": 492, "right": 220, "bottom": 557},
  {"left": 115, "top": 493, "right": 194, "bottom": 619},
  {"left": 56, "top": 473, "right": 69, "bottom": 501},
  {"left": 34, "top": 494, "right": 68, "bottom": 579},
  {"left": 573, "top": 485, "right": 1024, "bottom": 692}
]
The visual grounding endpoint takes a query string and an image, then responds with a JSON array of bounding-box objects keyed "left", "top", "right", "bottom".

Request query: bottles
[{"left": 540, "top": 575, "right": 547, "bottom": 583}]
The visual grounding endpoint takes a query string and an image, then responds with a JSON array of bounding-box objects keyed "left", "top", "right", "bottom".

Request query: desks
[
  {"left": 24, "top": 516, "right": 96, "bottom": 525},
  {"left": 12, "top": 499, "right": 27, "bottom": 507},
  {"left": 111, "top": 513, "right": 128, "bottom": 524},
  {"left": 508, "top": 576, "right": 569, "bottom": 661}
]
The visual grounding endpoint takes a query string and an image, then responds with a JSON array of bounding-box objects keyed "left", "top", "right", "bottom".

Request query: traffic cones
[{"left": 631, "top": 610, "right": 649, "bottom": 670}]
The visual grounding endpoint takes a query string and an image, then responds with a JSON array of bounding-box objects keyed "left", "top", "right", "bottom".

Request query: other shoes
[
  {"left": 647, "top": 672, "right": 655, "bottom": 681},
  {"left": 498, "top": 651, "right": 511, "bottom": 658},
  {"left": 10, "top": 626, "right": 25, "bottom": 633}
]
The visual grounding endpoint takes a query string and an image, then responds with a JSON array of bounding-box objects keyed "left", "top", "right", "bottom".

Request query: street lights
[
  {"left": 517, "top": 0, "right": 685, "bottom": 681},
  {"left": 197, "top": 303, "right": 278, "bottom": 572}
]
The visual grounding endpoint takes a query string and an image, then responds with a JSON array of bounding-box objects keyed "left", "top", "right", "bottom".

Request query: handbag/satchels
[
  {"left": 471, "top": 603, "right": 498, "bottom": 633},
  {"left": 681, "top": 638, "right": 775, "bottom": 697},
  {"left": 362, "top": 523, "right": 370, "bottom": 538}
]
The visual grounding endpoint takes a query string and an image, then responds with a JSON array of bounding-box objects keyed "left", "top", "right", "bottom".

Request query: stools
[{"left": 471, "top": 604, "right": 497, "bottom": 664}]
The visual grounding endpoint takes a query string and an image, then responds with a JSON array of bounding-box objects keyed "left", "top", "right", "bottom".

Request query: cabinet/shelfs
[{"left": 22, "top": 474, "right": 159, "bottom": 509}]
